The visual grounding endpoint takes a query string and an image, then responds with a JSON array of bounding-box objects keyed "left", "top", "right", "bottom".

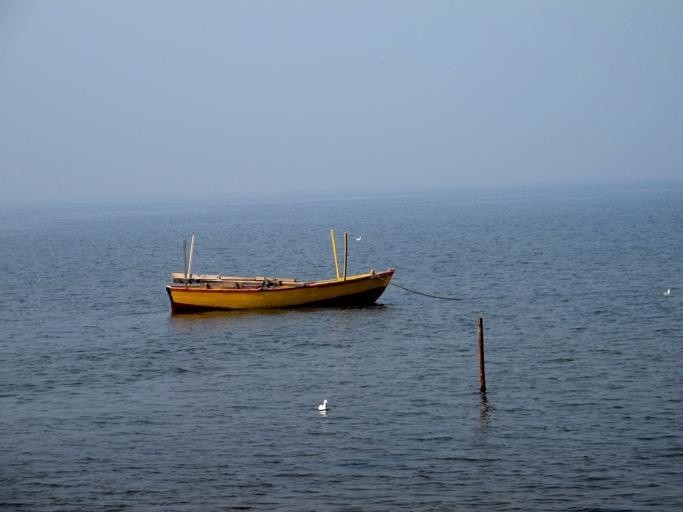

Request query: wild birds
[
  {"left": 662, "top": 288, "right": 671, "bottom": 296},
  {"left": 317, "top": 399, "right": 328, "bottom": 411},
  {"left": 354, "top": 236, "right": 361, "bottom": 241}
]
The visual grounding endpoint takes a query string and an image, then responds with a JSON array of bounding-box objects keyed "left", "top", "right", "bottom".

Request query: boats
[{"left": 165, "top": 228, "right": 395, "bottom": 315}]
